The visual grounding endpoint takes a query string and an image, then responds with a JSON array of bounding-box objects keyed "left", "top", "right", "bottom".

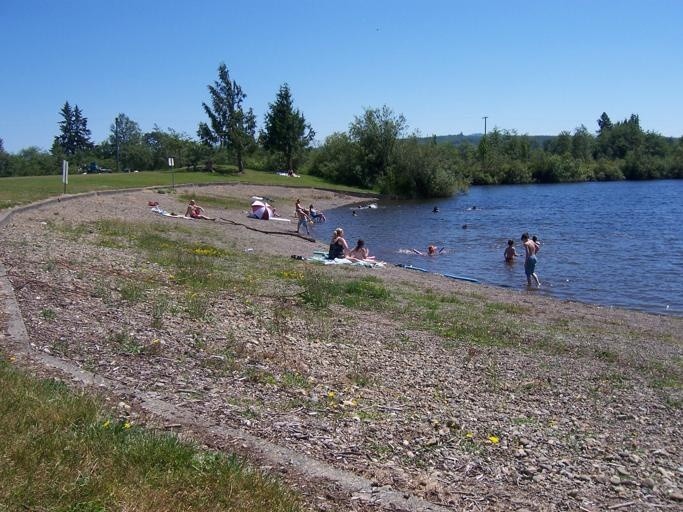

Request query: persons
[
  {"left": 520, "top": 232, "right": 540, "bottom": 288},
  {"left": 349, "top": 240, "right": 369, "bottom": 261},
  {"left": 185, "top": 200, "right": 217, "bottom": 221},
  {"left": 294, "top": 198, "right": 326, "bottom": 235},
  {"left": 327, "top": 228, "right": 349, "bottom": 260},
  {"left": 352, "top": 210, "right": 358, "bottom": 217},
  {"left": 413, "top": 246, "right": 446, "bottom": 255},
  {"left": 504, "top": 239, "right": 523, "bottom": 263},
  {"left": 531, "top": 235, "right": 540, "bottom": 246},
  {"left": 432, "top": 207, "right": 440, "bottom": 212},
  {"left": 273, "top": 208, "right": 279, "bottom": 218},
  {"left": 287, "top": 168, "right": 294, "bottom": 176}
]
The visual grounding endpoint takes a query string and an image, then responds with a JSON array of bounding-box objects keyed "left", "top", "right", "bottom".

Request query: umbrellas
[{"left": 252, "top": 200, "right": 275, "bottom": 219}]
